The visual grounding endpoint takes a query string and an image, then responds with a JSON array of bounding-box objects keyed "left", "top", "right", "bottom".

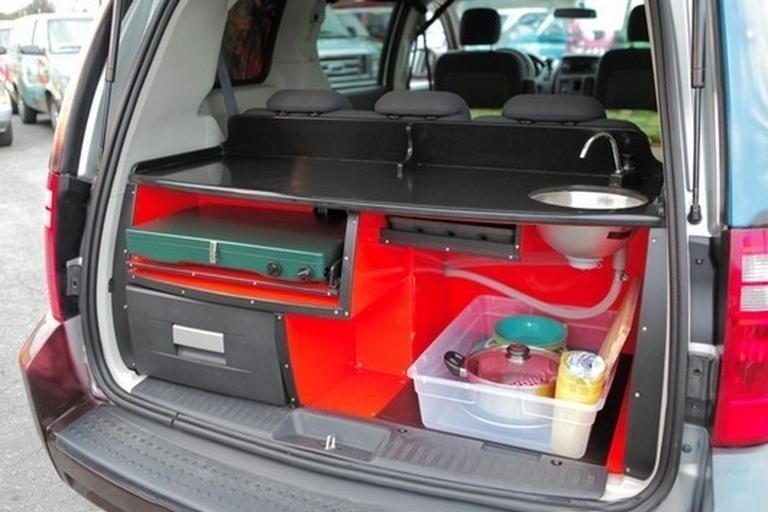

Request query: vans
[{"left": 0, "top": 20, "right": 11, "bottom": 78}]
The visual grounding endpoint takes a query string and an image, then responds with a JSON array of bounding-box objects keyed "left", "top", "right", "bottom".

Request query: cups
[{"left": 551, "top": 352, "right": 604, "bottom": 429}]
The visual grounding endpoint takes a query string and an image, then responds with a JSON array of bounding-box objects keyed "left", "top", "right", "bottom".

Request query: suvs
[{"left": 5, "top": 12, "right": 100, "bottom": 131}]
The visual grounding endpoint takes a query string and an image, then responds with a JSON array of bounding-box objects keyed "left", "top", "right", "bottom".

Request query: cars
[
  {"left": 16, "top": 0, "right": 768, "bottom": 512},
  {"left": 0, "top": 77, "right": 15, "bottom": 147},
  {"left": 469, "top": 1, "right": 641, "bottom": 60},
  {"left": 315, "top": 4, "right": 383, "bottom": 102}
]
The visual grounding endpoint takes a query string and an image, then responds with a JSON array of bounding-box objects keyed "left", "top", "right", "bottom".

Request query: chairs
[
  {"left": 243, "top": 85, "right": 358, "bottom": 120},
  {"left": 434, "top": 7, "right": 539, "bottom": 108},
  {"left": 498, "top": 83, "right": 614, "bottom": 126},
  {"left": 364, "top": 83, "right": 479, "bottom": 126},
  {"left": 592, "top": 5, "right": 655, "bottom": 109}
]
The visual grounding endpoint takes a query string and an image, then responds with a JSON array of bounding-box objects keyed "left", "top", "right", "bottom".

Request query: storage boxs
[{"left": 405, "top": 295, "right": 628, "bottom": 462}]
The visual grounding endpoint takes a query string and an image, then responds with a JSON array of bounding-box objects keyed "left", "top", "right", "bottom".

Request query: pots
[{"left": 442, "top": 342, "right": 563, "bottom": 426}]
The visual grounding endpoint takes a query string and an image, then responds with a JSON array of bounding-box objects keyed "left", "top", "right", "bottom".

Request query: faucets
[{"left": 580, "top": 131, "right": 622, "bottom": 169}]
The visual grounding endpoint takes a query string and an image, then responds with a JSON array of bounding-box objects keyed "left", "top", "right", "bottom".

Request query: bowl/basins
[{"left": 493, "top": 315, "right": 568, "bottom": 351}]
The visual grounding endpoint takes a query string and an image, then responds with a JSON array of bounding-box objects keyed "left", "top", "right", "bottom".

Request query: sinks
[{"left": 531, "top": 186, "right": 647, "bottom": 210}]
